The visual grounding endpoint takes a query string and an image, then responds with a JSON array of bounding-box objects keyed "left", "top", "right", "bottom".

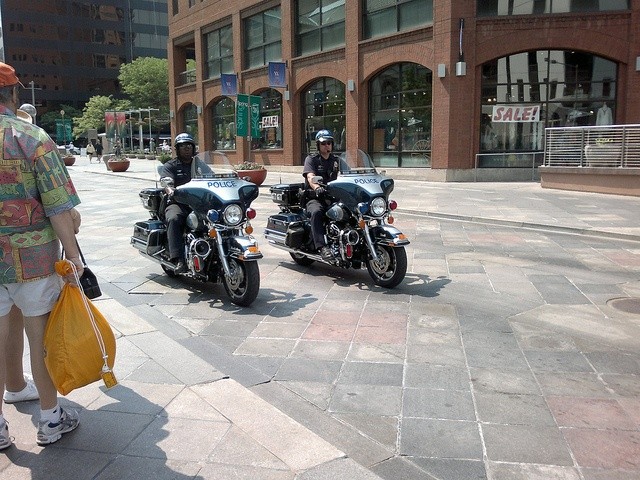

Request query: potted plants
[
  {"left": 234, "top": 161, "right": 267, "bottom": 186},
  {"left": 158, "top": 152, "right": 173, "bottom": 175},
  {"left": 62, "top": 154, "right": 75, "bottom": 166},
  {"left": 584, "top": 138, "right": 621, "bottom": 166},
  {"left": 107, "top": 153, "right": 131, "bottom": 173}
]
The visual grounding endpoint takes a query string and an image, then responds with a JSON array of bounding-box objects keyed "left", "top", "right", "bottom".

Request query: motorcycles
[
  {"left": 129, "top": 150, "right": 263, "bottom": 307},
  {"left": 263, "top": 148, "right": 410, "bottom": 288}
]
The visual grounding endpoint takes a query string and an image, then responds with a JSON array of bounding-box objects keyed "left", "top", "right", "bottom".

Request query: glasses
[
  {"left": 178, "top": 143, "right": 194, "bottom": 150},
  {"left": 320, "top": 140, "right": 332, "bottom": 145}
]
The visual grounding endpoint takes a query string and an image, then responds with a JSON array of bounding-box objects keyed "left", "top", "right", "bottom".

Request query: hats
[{"left": 0, "top": 61, "right": 25, "bottom": 91}]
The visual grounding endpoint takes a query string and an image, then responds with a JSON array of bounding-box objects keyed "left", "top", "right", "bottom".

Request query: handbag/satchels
[
  {"left": 43, "top": 261, "right": 118, "bottom": 396},
  {"left": 80, "top": 267, "right": 102, "bottom": 299}
]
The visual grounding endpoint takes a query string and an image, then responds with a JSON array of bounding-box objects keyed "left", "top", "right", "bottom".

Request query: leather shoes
[
  {"left": 173, "top": 258, "right": 188, "bottom": 273},
  {"left": 317, "top": 245, "right": 335, "bottom": 261}
]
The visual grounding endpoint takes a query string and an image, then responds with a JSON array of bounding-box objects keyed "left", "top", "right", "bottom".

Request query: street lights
[
  {"left": 60, "top": 110, "right": 68, "bottom": 155},
  {"left": 544, "top": 57, "right": 579, "bottom": 127},
  {"left": 15, "top": 76, "right": 36, "bottom": 125}
]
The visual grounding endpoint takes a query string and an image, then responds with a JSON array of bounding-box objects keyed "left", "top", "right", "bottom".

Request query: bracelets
[{"left": 314, "top": 186, "right": 320, "bottom": 192}]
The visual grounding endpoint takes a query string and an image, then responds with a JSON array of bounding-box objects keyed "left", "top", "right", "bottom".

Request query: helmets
[
  {"left": 315, "top": 129, "right": 335, "bottom": 152},
  {"left": 174, "top": 133, "right": 196, "bottom": 156}
]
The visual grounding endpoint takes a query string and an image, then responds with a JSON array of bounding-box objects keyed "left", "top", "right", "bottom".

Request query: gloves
[
  {"left": 168, "top": 191, "right": 176, "bottom": 203},
  {"left": 314, "top": 187, "right": 327, "bottom": 197}
]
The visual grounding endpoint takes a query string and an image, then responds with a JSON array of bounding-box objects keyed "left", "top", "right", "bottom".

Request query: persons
[
  {"left": 1, "top": 64, "right": 85, "bottom": 450},
  {"left": 94, "top": 140, "right": 103, "bottom": 163},
  {"left": 95, "top": 139, "right": 102, "bottom": 163},
  {"left": 158, "top": 132, "right": 215, "bottom": 273},
  {"left": 113, "top": 141, "right": 121, "bottom": 159},
  {"left": 69, "top": 142, "right": 73, "bottom": 155},
  {"left": 86, "top": 141, "right": 96, "bottom": 164},
  {"left": 3, "top": 192, "right": 82, "bottom": 411},
  {"left": 304, "top": 128, "right": 352, "bottom": 260}
]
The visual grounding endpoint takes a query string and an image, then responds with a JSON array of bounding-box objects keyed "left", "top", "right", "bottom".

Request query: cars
[{"left": 61, "top": 145, "right": 80, "bottom": 155}]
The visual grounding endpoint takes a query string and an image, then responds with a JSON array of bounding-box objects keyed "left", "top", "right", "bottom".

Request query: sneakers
[
  {"left": 36, "top": 407, "right": 80, "bottom": 445},
  {"left": 3, "top": 372, "right": 36, "bottom": 391},
  {"left": 0, "top": 418, "right": 12, "bottom": 450},
  {"left": 3, "top": 382, "right": 40, "bottom": 403}
]
